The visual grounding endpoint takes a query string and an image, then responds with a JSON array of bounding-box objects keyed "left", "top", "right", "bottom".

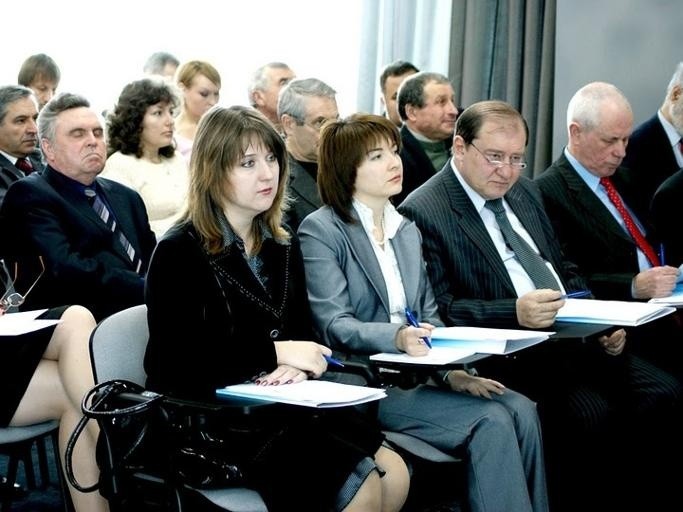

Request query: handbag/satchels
[{"left": 65, "top": 378, "right": 244, "bottom": 491}]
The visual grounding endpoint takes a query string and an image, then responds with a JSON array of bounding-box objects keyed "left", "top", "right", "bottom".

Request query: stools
[{"left": 0, "top": 421, "right": 60, "bottom": 507}]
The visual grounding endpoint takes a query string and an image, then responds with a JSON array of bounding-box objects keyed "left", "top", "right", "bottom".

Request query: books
[{"left": 225, "top": 381, "right": 390, "bottom": 408}]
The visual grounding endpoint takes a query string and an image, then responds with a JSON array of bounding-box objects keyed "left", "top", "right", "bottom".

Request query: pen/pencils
[
  {"left": 554, "top": 289, "right": 591, "bottom": 301},
  {"left": 323, "top": 354, "right": 344, "bottom": 367},
  {"left": 659, "top": 243, "right": 664, "bottom": 266},
  {"left": 405, "top": 308, "right": 432, "bottom": 349}
]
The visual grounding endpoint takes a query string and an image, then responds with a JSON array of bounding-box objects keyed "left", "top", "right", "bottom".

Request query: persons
[
  {"left": 247, "top": 63, "right": 298, "bottom": 138},
  {"left": 2, "top": 84, "right": 45, "bottom": 202},
  {"left": 97, "top": 79, "right": 190, "bottom": 242},
  {"left": 532, "top": 82, "right": 682, "bottom": 406},
  {"left": 18, "top": 53, "right": 61, "bottom": 111},
  {"left": 143, "top": 104, "right": 410, "bottom": 511},
  {"left": 379, "top": 59, "right": 419, "bottom": 130},
  {"left": 297, "top": 111, "right": 549, "bottom": 510},
  {"left": 392, "top": 71, "right": 464, "bottom": 205},
  {"left": 144, "top": 52, "right": 179, "bottom": 84},
  {"left": 2, "top": 92, "right": 157, "bottom": 325},
  {"left": 174, "top": 60, "right": 221, "bottom": 158},
  {"left": 277, "top": 78, "right": 339, "bottom": 233},
  {"left": 609, "top": 67, "right": 682, "bottom": 219},
  {"left": 644, "top": 168, "right": 682, "bottom": 268},
  {"left": 2, "top": 305, "right": 110, "bottom": 511}
]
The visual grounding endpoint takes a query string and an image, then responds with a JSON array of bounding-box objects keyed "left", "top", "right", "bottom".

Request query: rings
[{"left": 418, "top": 340, "right": 421, "bottom": 345}]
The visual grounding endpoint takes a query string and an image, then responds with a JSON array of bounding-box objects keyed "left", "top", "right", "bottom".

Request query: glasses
[
  {"left": 0, "top": 256, "right": 45, "bottom": 313},
  {"left": 471, "top": 142, "right": 527, "bottom": 170}
]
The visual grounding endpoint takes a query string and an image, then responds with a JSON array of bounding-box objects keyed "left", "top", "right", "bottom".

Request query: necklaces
[
  {"left": 377, "top": 239, "right": 384, "bottom": 245},
  {"left": 210, "top": 243, "right": 290, "bottom": 318}
]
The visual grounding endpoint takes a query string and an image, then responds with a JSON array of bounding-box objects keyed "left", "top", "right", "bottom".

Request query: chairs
[
  {"left": 323, "top": 359, "right": 469, "bottom": 512},
  {"left": 88, "top": 303, "right": 265, "bottom": 508}
]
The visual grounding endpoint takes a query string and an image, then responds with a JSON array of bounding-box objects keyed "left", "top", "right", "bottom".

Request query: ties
[
  {"left": 84, "top": 187, "right": 142, "bottom": 274},
  {"left": 15, "top": 158, "right": 33, "bottom": 176},
  {"left": 600, "top": 177, "right": 660, "bottom": 266},
  {"left": 484, "top": 198, "right": 560, "bottom": 291}
]
